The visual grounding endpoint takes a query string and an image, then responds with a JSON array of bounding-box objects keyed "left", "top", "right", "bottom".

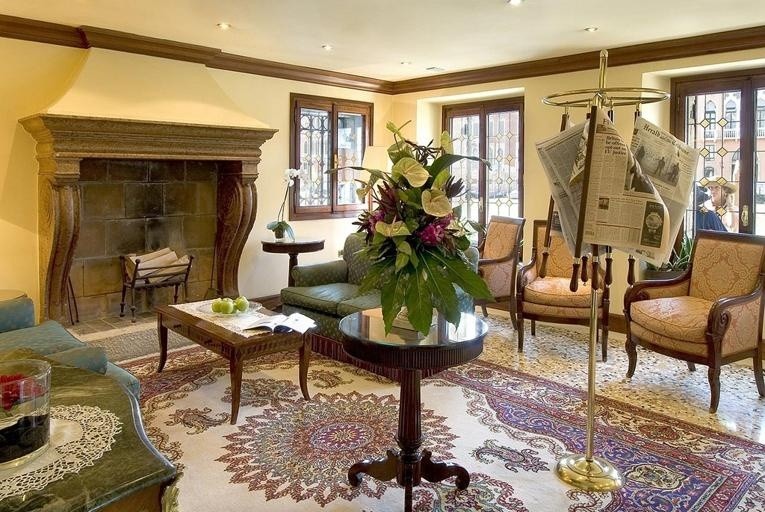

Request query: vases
[{"left": 273, "top": 226, "right": 285, "bottom": 242}]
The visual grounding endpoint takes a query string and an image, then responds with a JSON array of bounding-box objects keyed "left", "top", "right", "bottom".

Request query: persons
[
  {"left": 654, "top": 156, "right": 665, "bottom": 176},
  {"left": 636, "top": 145, "right": 645, "bottom": 162},
  {"left": 702, "top": 176, "right": 738, "bottom": 233},
  {"left": 668, "top": 162, "right": 679, "bottom": 183}
]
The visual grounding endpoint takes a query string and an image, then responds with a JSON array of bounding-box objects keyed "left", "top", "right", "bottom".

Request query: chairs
[
  {"left": 623, "top": 229, "right": 765, "bottom": 414},
  {"left": 516, "top": 218, "right": 611, "bottom": 362},
  {"left": 475, "top": 214, "right": 526, "bottom": 330}
]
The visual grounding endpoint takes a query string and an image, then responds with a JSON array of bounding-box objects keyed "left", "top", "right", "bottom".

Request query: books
[{"left": 241, "top": 310, "right": 317, "bottom": 336}]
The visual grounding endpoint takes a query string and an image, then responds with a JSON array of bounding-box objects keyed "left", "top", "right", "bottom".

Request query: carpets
[
  {"left": 81, "top": 326, "right": 194, "bottom": 362},
  {"left": 114, "top": 345, "right": 764, "bottom": 512}
]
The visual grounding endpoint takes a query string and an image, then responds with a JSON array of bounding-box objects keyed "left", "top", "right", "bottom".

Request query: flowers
[
  {"left": 324, "top": 120, "right": 497, "bottom": 341},
  {"left": 266, "top": 164, "right": 305, "bottom": 242}
]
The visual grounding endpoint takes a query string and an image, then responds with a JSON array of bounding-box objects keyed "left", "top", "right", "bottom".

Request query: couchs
[
  {"left": 280, "top": 233, "right": 478, "bottom": 385},
  {"left": 0, "top": 294, "right": 139, "bottom": 394}
]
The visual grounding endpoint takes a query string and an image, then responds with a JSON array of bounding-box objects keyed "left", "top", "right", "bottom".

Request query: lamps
[
  {"left": 507, "top": 0, "right": 524, "bottom": 5},
  {"left": 218, "top": 24, "right": 231, "bottom": 29},
  {"left": 584, "top": 27, "right": 598, "bottom": 33},
  {"left": 323, "top": 44, "right": 333, "bottom": 50}
]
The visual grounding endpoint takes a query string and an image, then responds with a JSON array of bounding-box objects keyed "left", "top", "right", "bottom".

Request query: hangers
[{"left": 538, "top": 45, "right": 674, "bottom": 492}]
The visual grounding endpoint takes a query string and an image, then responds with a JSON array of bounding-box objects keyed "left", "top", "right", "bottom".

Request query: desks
[
  {"left": 339, "top": 306, "right": 487, "bottom": 512},
  {"left": 154, "top": 296, "right": 320, "bottom": 424},
  {"left": 262, "top": 237, "right": 325, "bottom": 292},
  {"left": 0, "top": 346, "right": 180, "bottom": 512}
]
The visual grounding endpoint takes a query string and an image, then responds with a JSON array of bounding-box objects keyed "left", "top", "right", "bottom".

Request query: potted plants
[{"left": 644, "top": 228, "right": 693, "bottom": 280}]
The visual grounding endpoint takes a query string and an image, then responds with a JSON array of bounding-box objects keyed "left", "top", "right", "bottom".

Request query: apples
[
  {"left": 220, "top": 299, "right": 233, "bottom": 313},
  {"left": 212, "top": 298, "right": 222, "bottom": 312},
  {"left": 234, "top": 296, "right": 249, "bottom": 312},
  {"left": 232, "top": 302, "right": 236, "bottom": 314},
  {"left": 223, "top": 298, "right": 233, "bottom": 303}
]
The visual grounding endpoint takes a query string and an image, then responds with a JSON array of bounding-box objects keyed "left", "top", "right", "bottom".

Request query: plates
[{"left": 196, "top": 301, "right": 263, "bottom": 317}]
